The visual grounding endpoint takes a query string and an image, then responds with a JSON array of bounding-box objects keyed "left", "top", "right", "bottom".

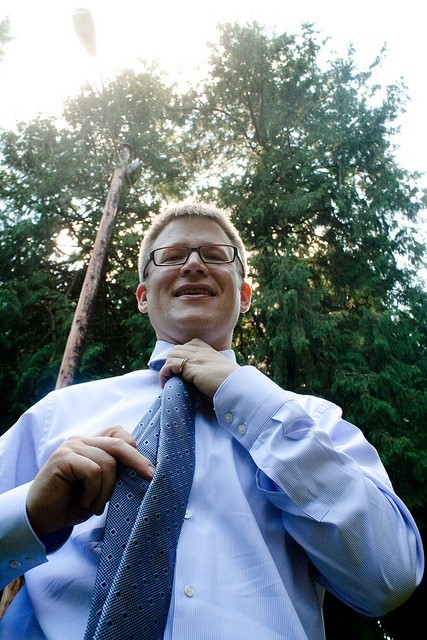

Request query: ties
[{"left": 85, "top": 357, "right": 195, "bottom": 639}]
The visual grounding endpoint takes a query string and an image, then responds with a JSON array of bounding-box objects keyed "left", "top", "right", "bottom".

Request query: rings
[{"left": 176, "top": 358, "right": 188, "bottom": 374}]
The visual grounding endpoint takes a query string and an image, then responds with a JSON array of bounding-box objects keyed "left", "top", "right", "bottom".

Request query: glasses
[{"left": 142, "top": 244, "right": 245, "bottom": 278}]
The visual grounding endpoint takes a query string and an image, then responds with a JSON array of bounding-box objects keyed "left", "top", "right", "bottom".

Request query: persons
[{"left": 0, "top": 200, "right": 425, "bottom": 639}]
